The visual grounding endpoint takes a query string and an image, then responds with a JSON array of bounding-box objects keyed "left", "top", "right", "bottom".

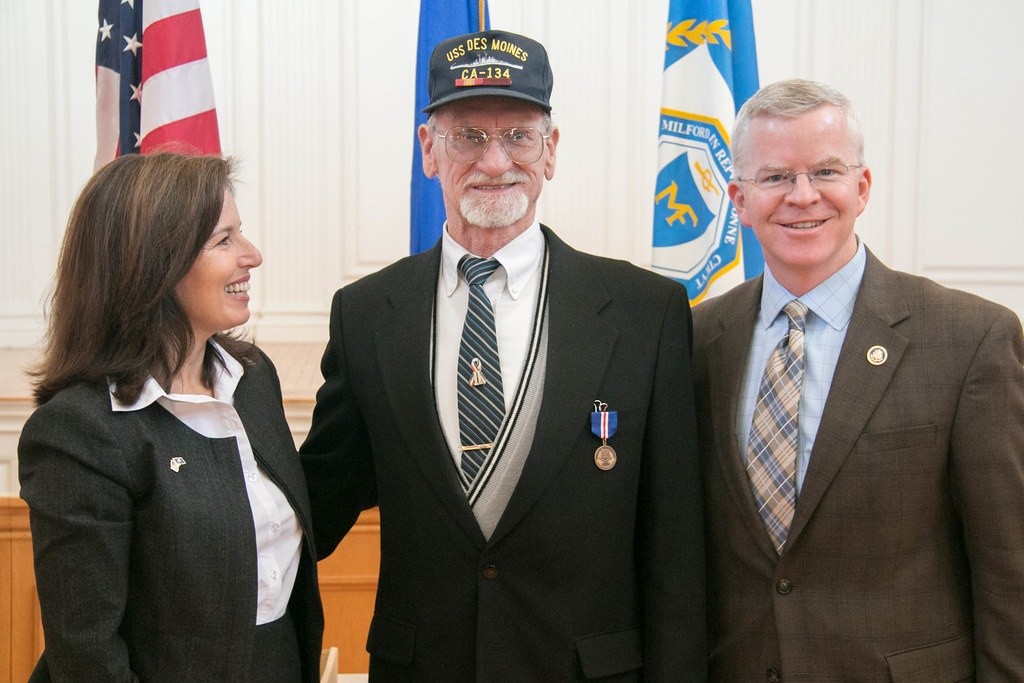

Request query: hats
[{"left": 418, "top": 28, "right": 555, "bottom": 114}]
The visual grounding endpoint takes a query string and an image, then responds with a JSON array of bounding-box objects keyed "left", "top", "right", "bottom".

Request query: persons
[
  {"left": 691, "top": 81, "right": 1024, "bottom": 683},
  {"left": 17, "top": 151, "right": 325, "bottom": 683},
  {"left": 298, "top": 30, "right": 707, "bottom": 682}
]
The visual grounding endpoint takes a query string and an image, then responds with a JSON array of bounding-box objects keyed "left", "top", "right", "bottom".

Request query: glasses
[
  {"left": 737, "top": 160, "right": 867, "bottom": 195},
  {"left": 428, "top": 123, "right": 553, "bottom": 166}
]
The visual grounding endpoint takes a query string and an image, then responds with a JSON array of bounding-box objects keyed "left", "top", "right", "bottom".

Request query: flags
[
  {"left": 410, "top": 0, "right": 491, "bottom": 255},
  {"left": 96, "top": 1, "right": 223, "bottom": 172},
  {"left": 651, "top": 0, "right": 764, "bottom": 306}
]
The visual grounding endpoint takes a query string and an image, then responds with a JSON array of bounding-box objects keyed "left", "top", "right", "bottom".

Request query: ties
[
  {"left": 745, "top": 299, "right": 810, "bottom": 557},
  {"left": 457, "top": 255, "right": 507, "bottom": 484}
]
